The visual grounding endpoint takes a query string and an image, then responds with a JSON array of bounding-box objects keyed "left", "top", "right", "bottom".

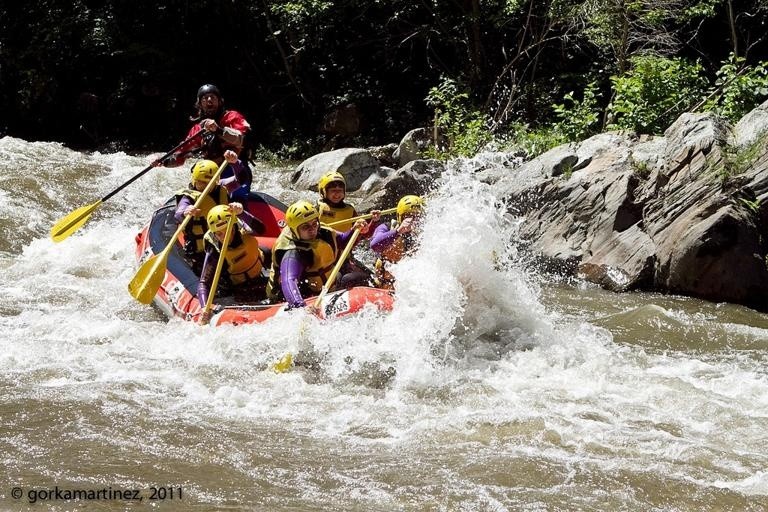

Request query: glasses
[{"left": 301, "top": 221, "right": 318, "bottom": 231}]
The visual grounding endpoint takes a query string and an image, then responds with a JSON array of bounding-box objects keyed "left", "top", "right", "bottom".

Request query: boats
[{"left": 136, "top": 192, "right": 396, "bottom": 328}]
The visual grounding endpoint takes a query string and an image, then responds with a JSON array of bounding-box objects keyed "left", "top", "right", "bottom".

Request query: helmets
[
  {"left": 397, "top": 195, "right": 426, "bottom": 224},
  {"left": 319, "top": 172, "right": 346, "bottom": 199},
  {"left": 285, "top": 201, "right": 320, "bottom": 241},
  {"left": 196, "top": 84, "right": 224, "bottom": 106},
  {"left": 207, "top": 204, "right": 238, "bottom": 232},
  {"left": 191, "top": 160, "right": 221, "bottom": 188}
]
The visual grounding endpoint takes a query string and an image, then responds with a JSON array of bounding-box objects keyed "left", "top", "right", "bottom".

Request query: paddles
[
  {"left": 51, "top": 129, "right": 206, "bottom": 243},
  {"left": 270, "top": 226, "right": 361, "bottom": 373},
  {"left": 128, "top": 159, "right": 228, "bottom": 304}
]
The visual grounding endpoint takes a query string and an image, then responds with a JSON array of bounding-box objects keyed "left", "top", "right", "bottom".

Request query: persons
[
  {"left": 150, "top": 84, "right": 252, "bottom": 193},
  {"left": 266, "top": 199, "right": 368, "bottom": 309},
  {"left": 174, "top": 150, "right": 272, "bottom": 277},
  {"left": 368, "top": 195, "right": 425, "bottom": 289},
  {"left": 314, "top": 171, "right": 381, "bottom": 233},
  {"left": 196, "top": 202, "right": 269, "bottom": 326}
]
